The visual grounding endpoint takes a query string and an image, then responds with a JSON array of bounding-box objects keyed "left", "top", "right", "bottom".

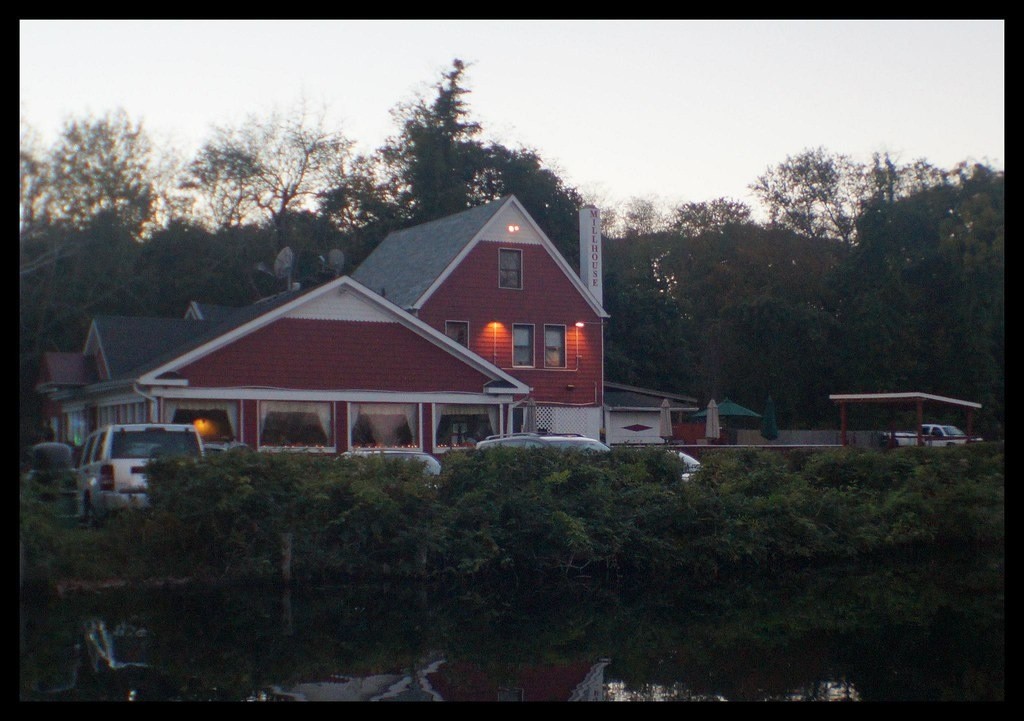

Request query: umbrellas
[
  {"left": 659, "top": 399, "right": 673, "bottom": 445},
  {"left": 691, "top": 396, "right": 762, "bottom": 445},
  {"left": 705, "top": 399, "right": 720, "bottom": 446},
  {"left": 522, "top": 398, "right": 539, "bottom": 434}
]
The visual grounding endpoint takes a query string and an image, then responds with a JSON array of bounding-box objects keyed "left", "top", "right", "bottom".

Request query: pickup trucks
[
  {"left": 886, "top": 423, "right": 986, "bottom": 449},
  {"left": 80, "top": 421, "right": 205, "bottom": 524}
]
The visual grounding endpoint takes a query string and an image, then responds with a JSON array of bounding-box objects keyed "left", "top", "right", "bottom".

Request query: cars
[
  {"left": 23, "top": 443, "right": 89, "bottom": 520},
  {"left": 335, "top": 449, "right": 447, "bottom": 502},
  {"left": 472, "top": 436, "right": 610, "bottom": 482},
  {"left": 652, "top": 447, "right": 704, "bottom": 488}
]
